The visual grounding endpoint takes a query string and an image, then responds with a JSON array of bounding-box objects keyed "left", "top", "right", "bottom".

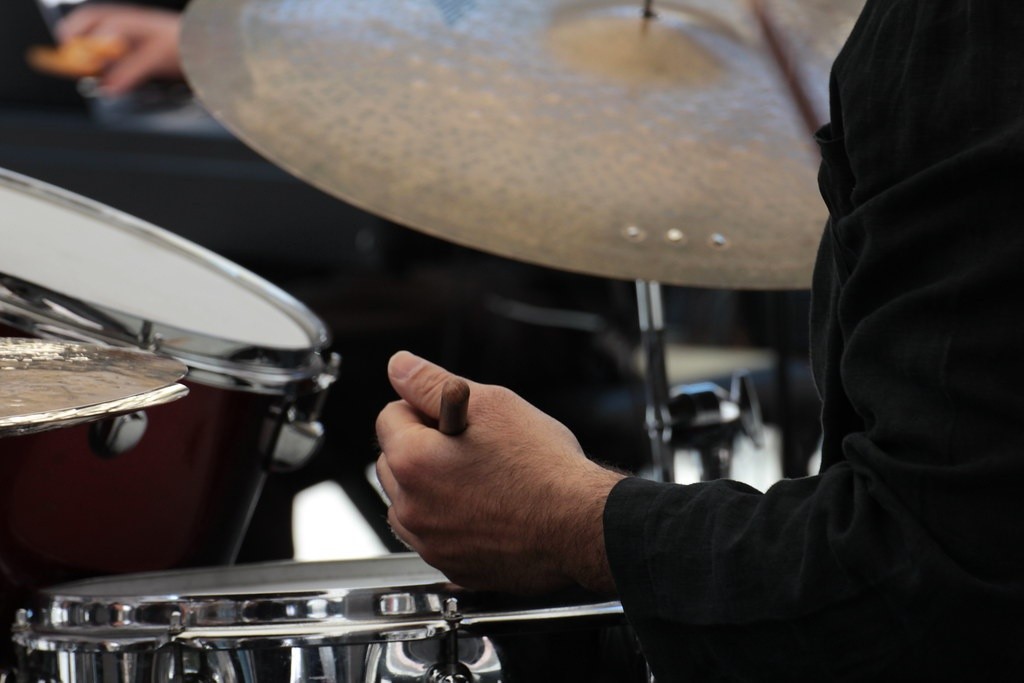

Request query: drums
[
  {"left": 7, "top": 548, "right": 506, "bottom": 683},
  {"left": 1, "top": 163, "right": 345, "bottom": 622}
]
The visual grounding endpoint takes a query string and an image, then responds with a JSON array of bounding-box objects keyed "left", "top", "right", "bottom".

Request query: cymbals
[
  {"left": 179, "top": 0, "right": 877, "bottom": 294},
  {"left": 0, "top": 332, "right": 191, "bottom": 436}
]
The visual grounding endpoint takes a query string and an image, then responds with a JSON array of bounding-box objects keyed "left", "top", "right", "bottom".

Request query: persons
[
  {"left": 33, "top": 1, "right": 178, "bottom": 105},
  {"left": 369, "top": 1, "right": 1023, "bottom": 683}
]
